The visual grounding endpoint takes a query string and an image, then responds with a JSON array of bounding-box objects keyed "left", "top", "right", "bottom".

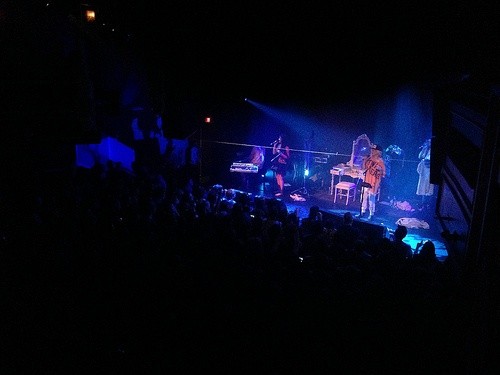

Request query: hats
[{"left": 370, "top": 143, "right": 383, "bottom": 152}]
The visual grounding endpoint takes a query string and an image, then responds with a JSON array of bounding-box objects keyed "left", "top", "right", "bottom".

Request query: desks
[{"left": 330, "top": 165, "right": 380, "bottom": 202}]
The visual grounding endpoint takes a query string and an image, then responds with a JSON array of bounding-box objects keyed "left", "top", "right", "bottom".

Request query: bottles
[
  {"left": 393, "top": 196, "right": 396, "bottom": 200},
  {"left": 388, "top": 196, "right": 391, "bottom": 202}
]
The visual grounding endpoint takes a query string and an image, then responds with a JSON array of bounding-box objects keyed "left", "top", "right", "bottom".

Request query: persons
[
  {"left": 141, "top": 180, "right": 437, "bottom": 274},
  {"left": 415, "top": 148, "right": 434, "bottom": 211},
  {"left": 355, "top": 142, "right": 385, "bottom": 220},
  {"left": 253, "top": 146, "right": 265, "bottom": 196},
  {"left": 272, "top": 135, "right": 290, "bottom": 197}
]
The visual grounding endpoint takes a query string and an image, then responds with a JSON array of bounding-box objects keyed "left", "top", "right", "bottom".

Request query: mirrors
[{"left": 351, "top": 133, "right": 374, "bottom": 169}]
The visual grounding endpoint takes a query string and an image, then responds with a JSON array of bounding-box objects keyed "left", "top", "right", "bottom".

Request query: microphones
[{"left": 270, "top": 140, "right": 277, "bottom": 145}]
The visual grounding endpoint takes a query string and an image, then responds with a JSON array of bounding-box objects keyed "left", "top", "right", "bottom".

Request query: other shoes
[
  {"left": 418, "top": 203, "right": 424, "bottom": 210},
  {"left": 427, "top": 203, "right": 432, "bottom": 209},
  {"left": 368, "top": 215, "right": 374, "bottom": 221},
  {"left": 355, "top": 213, "right": 364, "bottom": 218},
  {"left": 275, "top": 192, "right": 283, "bottom": 197}
]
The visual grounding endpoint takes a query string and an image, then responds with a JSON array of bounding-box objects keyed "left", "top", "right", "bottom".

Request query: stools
[{"left": 334, "top": 182, "right": 357, "bottom": 206}]
